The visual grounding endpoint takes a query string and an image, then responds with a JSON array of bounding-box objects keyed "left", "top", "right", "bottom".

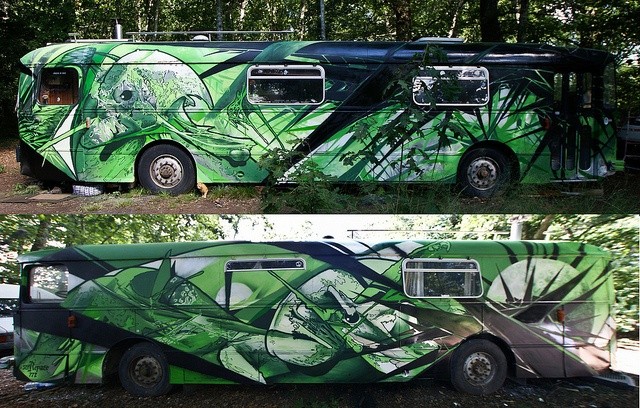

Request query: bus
[
  {"left": 13, "top": 18, "right": 625, "bottom": 200},
  {"left": 13, "top": 238, "right": 618, "bottom": 396}
]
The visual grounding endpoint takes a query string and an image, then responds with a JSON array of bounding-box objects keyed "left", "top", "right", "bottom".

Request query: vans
[
  {"left": 0, "top": 283, "right": 20, "bottom": 358},
  {"left": 617, "top": 116, "right": 640, "bottom": 146}
]
